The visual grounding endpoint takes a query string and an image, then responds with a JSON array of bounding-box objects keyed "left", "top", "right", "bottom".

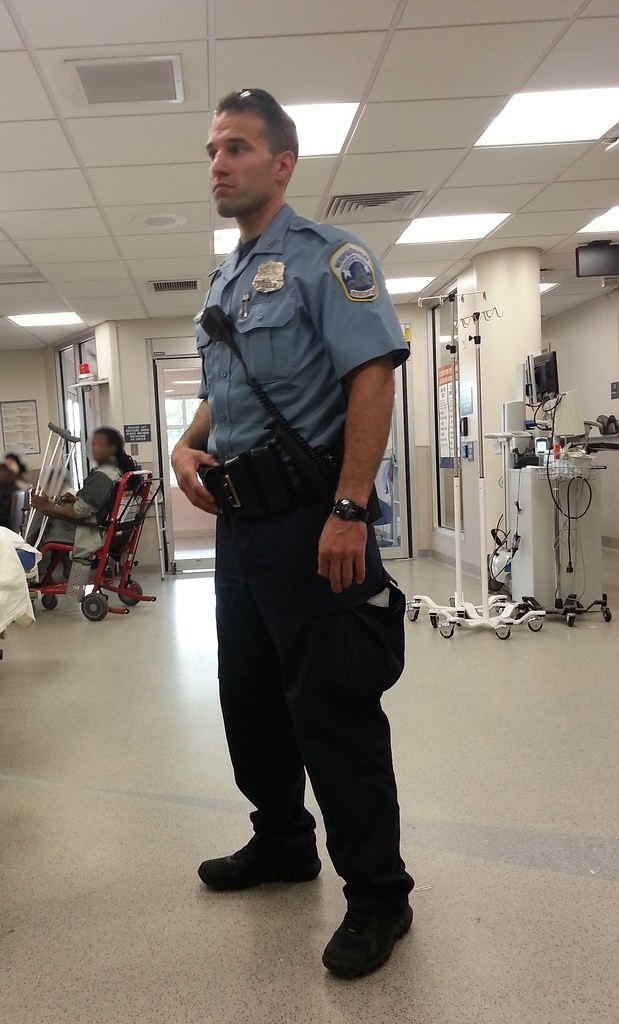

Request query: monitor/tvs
[{"left": 533, "top": 351, "right": 559, "bottom": 402}]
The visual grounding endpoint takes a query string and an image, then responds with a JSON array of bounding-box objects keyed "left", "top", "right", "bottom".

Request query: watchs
[{"left": 332, "top": 498, "right": 368, "bottom": 522}]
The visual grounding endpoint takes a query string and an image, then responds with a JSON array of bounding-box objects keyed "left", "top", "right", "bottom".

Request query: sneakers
[
  {"left": 198, "top": 832, "right": 321, "bottom": 891},
  {"left": 322, "top": 896, "right": 413, "bottom": 977}
]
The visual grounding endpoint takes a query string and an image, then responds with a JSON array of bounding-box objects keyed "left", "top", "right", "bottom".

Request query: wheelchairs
[{"left": 26, "top": 467, "right": 160, "bottom": 622}]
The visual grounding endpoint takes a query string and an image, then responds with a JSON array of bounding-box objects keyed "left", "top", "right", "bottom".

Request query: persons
[
  {"left": 0, "top": 454, "right": 32, "bottom": 527},
  {"left": 27, "top": 427, "right": 144, "bottom": 588},
  {"left": 171, "top": 88, "right": 413, "bottom": 978}
]
